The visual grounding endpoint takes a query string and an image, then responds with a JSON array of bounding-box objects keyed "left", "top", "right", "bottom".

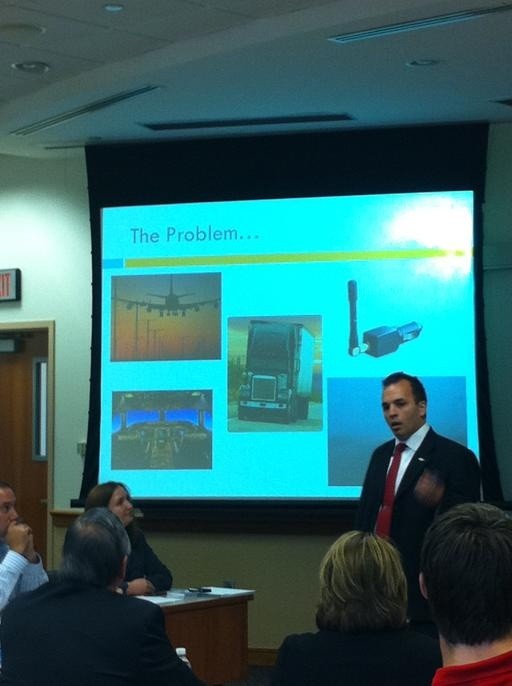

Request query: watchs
[{"left": 118, "top": 581, "right": 128, "bottom": 594}]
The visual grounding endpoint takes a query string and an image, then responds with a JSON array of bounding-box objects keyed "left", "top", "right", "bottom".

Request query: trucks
[{"left": 237, "top": 320, "right": 317, "bottom": 424}]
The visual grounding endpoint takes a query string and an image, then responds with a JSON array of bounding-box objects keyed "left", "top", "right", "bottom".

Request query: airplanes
[{"left": 111, "top": 275, "right": 219, "bottom": 318}]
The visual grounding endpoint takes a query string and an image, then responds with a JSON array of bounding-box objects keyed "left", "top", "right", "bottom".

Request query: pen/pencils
[{"left": 188, "top": 588, "right": 212, "bottom": 592}]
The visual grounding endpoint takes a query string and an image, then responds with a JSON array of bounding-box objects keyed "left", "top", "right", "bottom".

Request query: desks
[{"left": 132, "top": 587, "right": 255, "bottom": 686}]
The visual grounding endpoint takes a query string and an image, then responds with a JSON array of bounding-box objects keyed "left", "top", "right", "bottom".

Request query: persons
[
  {"left": 268, "top": 530, "right": 442, "bottom": 686},
  {"left": 85, "top": 482, "right": 172, "bottom": 596},
  {"left": 0, "top": 483, "right": 49, "bottom": 673},
  {"left": 0, "top": 506, "right": 208, "bottom": 686},
  {"left": 419, "top": 503, "right": 512, "bottom": 686},
  {"left": 353, "top": 373, "right": 480, "bottom": 638}
]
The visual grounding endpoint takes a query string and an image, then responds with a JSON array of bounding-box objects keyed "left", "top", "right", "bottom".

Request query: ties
[{"left": 375, "top": 444, "right": 407, "bottom": 537}]
